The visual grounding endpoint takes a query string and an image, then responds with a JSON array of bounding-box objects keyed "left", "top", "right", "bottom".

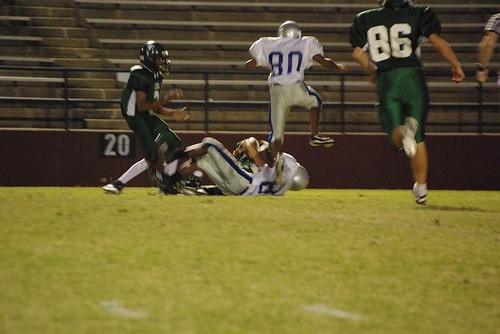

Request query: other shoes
[
  {"left": 412, "top": 181, "right": 427, "bottom": 204},
  {"left": 154, "top": 170, "right": 172, "bottom": 193},
  {"left": 402, "top": 116, "right": 420, "bottom": 158}
]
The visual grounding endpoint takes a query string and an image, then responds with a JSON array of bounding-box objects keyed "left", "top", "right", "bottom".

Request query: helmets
[
  {"left": 277, "top": 21, "right": 302, "bottom": 38},
  {"left": 138, "top": 40, "right": 172, "bottom": 78},
  {"left": 288, "top": 164, "right": 310, "bottom": 190}
]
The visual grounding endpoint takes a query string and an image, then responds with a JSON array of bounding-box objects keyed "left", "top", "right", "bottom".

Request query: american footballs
[{"left": 247, "top": 136, "right": 259, "bottom": 161}]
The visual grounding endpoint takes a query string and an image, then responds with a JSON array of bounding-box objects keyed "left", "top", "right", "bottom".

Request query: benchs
[{"left": 0, "top": 0, "right": 500, "bottom": 132}]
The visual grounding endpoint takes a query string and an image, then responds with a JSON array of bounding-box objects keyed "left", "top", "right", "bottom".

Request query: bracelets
[{"left": 477, "top": 65, "right": 488, "bottom": 71}]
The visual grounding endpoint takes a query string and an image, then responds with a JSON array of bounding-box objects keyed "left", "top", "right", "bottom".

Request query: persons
[
  {"left": 103, "top": 40, "right": 189, "bottom": 195},
  {"left": 476, "top": 12, "right": 500, "bottom": 87},
  {"left": 163, "top": 137, "right": 309, "bottom": 196},
  {"left": 246, "top": 20, "right": 347, "bottom": 147},
  {"left": 348, "top": 0, "right": 465, "bottom": 205}
]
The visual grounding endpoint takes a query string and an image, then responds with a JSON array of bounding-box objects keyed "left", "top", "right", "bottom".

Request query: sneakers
[
  {"left": 103, "top": 180, "right": 126, "bottom": 194},
  {"left": 308, "top": 133, "right": 335, "bottom": 148}
]
